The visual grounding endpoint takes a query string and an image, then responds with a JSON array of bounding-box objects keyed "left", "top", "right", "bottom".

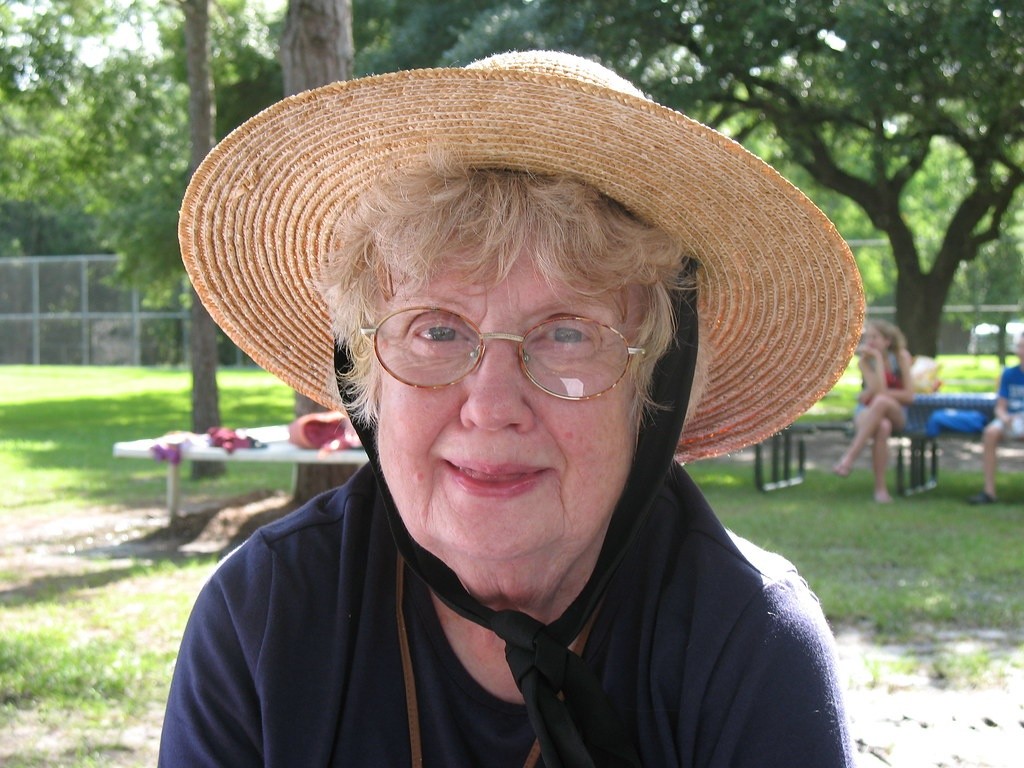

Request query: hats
[{"left": 180, "top": 48, "right": 864, "bottom": 462}]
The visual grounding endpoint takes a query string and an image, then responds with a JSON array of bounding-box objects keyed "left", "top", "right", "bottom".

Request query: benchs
[
  {"left": 755, "top": 394, "right": 1024, "bottom": 498},
  {"left": 113, "top": 425, "right": 370, "bottom": 531}
]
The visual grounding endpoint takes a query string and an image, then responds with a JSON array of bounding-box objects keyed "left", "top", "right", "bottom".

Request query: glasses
[{"left": 360, "top": 305, "right": 646, "bottom": 401}]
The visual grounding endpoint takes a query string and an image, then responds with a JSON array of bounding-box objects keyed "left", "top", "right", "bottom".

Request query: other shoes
[{"left": 967, "top": 491, "right": 997, "bottom": 505}]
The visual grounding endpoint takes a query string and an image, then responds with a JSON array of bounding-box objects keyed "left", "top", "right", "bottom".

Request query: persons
[
  {"left": 155, "top": 49, "right": 857, "bottom": 768},
  {"left": 833, "top": 316, "right": 914, "bottom": 503},
  {"left": 968, "top": 332, "right": 1024, "bottom": 505}
]
